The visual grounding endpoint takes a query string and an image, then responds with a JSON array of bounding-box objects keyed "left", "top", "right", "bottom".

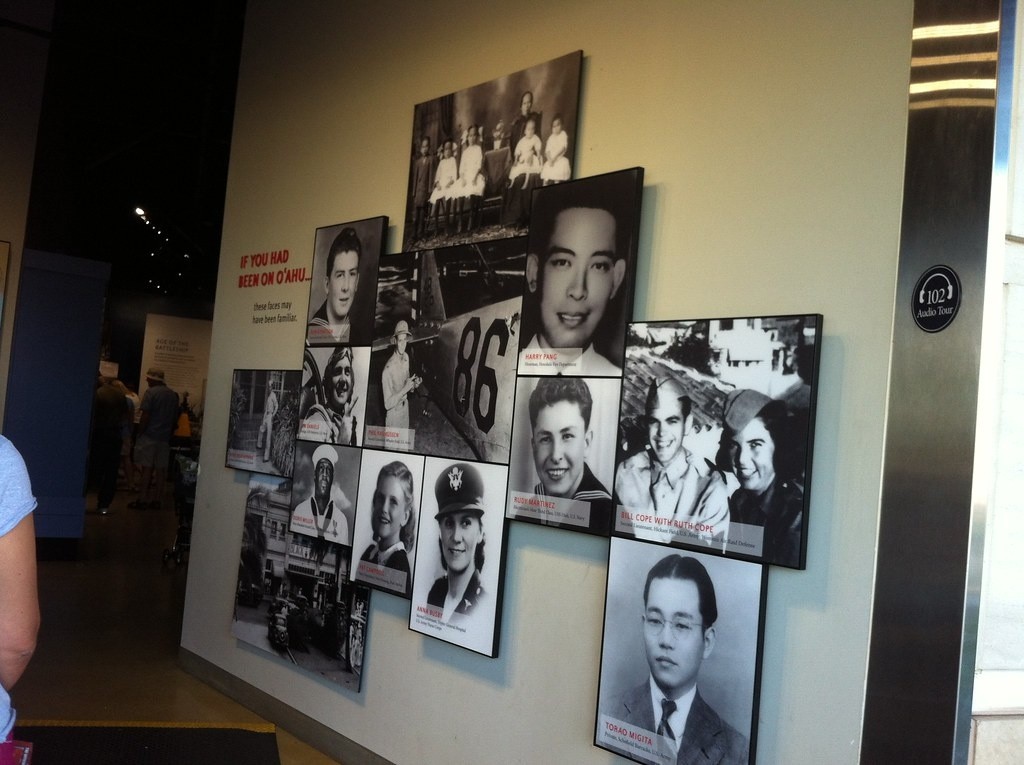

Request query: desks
[{"left": 13, "top": 719, "right": 280, "bottom": 765}]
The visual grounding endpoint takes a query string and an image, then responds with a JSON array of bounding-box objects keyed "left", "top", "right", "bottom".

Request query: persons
[
  {"left": 522, "top": 379, "right": 614, "bottom": 527},
  {"left": 611, "top": 555, "right": 755, "bottom": 764},
  {"left": 384, "top": 320, "right": 421, "bottom": 448},
  {"left": 0, "top": 434, "right": 40, "bottom": 765},
  {"left": 88, "top": 367, "right": 181, "bottom": 515},
  {"left": 355, "top": 462, "right": 416, "bottom": 586},
  {"left": 300, "top": 347, "right": 359, "bottom": 445},
  {"left": 291, "top": 445, "right": 350, "bottom": 547},
  {"left": 429, "top": 463, "right": 491, "bottom": 632},
  {"left": 617, "top": 371, "right": 806, "bottom": 555},
  {"left": 410, "top": 90, "right": 568, "bottom": 235},
  {"left": 526, "top": 199, "right": 630, "bottom": 377},
  {"left": 256, "top": 380, "right": 278, "bottom": 463},
  {"left": 307, "top": 227, "right": 363, "bottom": 343}
]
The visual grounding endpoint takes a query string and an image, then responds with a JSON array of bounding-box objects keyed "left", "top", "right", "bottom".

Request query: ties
[{"left": 657, "top": 700, "right": 676, "bottom": 739}]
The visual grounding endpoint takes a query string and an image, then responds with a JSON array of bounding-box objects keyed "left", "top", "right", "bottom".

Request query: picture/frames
[{"left": 222, "top": 165, "right": 823, "bottom": 765}]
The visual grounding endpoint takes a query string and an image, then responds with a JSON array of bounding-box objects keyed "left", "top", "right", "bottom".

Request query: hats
[
  {"left": 646, "top": 374, "right": 687, "bottom": 409},
  {"left": 434, "top": 463, "right": 485, "bottom": 516},
  {"left": 722, "top": 389, "right": 772, "bottom": 436},
  {"left": 144, "top": 368, "right": 164, "bottom": 383},
  {"left": 312, "top": 444, "right": 338, "bottom": 469},
  {"left": 390, "top": 320, "right": 413, "bottom": 346}
]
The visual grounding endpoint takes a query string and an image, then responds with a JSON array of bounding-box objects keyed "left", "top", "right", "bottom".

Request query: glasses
[{"left": 643, "top": 611, "right": 708, "bottom": 640}]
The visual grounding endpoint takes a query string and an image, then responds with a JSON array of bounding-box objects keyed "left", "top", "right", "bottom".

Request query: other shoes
[
  {"left": 96, "top": 506, "right": 108, "bottom": 515},
  {"left": 412, "top": 223, "right": 483, "bottom": 241},
  {"left": 128, "top": 499, "right": 151, "bottom": 510}
]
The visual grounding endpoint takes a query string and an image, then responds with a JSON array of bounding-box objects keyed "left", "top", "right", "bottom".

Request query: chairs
[{"left": 158, "top": 436, "right": 200, "bottom": 568}]
